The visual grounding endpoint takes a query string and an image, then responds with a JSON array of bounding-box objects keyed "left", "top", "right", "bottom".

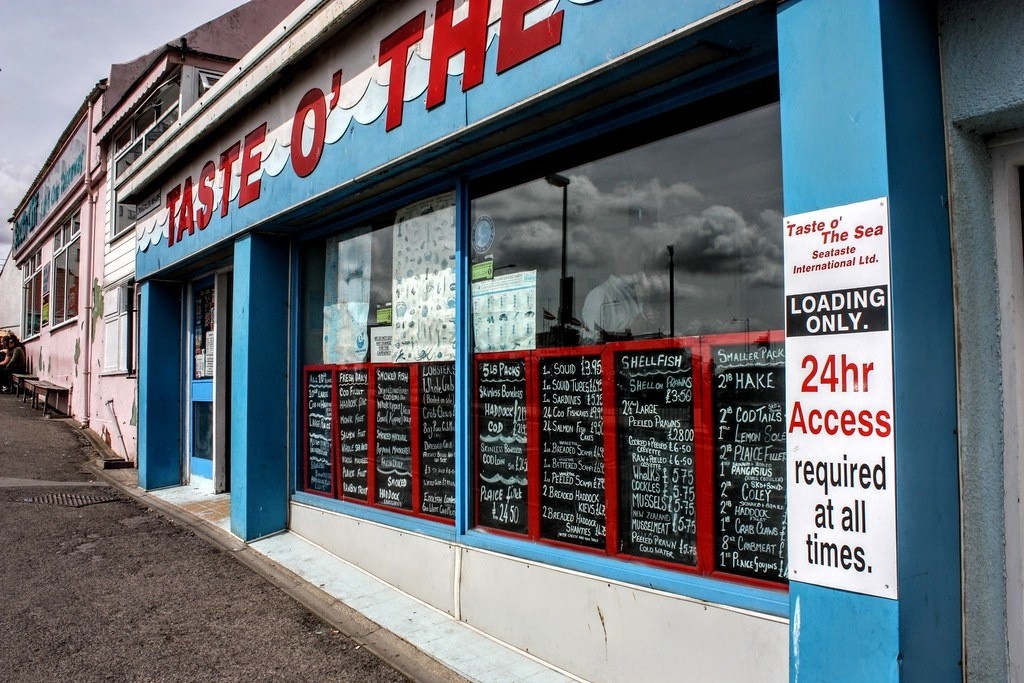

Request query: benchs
[{"left": 13, "top": 371, "right": 69, "bottom": 418}]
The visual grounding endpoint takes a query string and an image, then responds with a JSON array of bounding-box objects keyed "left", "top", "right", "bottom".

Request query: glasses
[{"left": 9, "top": 341, "right": 13, "bottom": 344}]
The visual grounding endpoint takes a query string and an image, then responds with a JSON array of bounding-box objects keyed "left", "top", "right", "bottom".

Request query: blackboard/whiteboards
[
  {"left": 334, "top": 362, "right": 371, "bottom": 506},
  {"left": 414, "top": 361, "right": 456, "bottom": 528},
  {"left": 610, "top": 336, "right": 703, "bottom": 577},
  {"left": 704, "top": 328, "right": 788, "bottom": 594},
  {"left": 531, "top": 342, "right": 609, "bottom": 559},
  {"left": 474, "top": 350, "right": 531, "bottom": 543},
  {"left": 302, "top": 364, "right": 337, "bottom": 500},
  {"left": 372, "top": 362, "right": 415, "bottom": 516}
]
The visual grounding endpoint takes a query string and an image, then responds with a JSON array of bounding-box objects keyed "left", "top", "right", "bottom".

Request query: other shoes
[{"left": 2, "top": 386, "right": 10, "bottom": 393}]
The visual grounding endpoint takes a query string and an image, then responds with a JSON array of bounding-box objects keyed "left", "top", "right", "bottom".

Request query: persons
[
  {"left": 0, "top": 333, "right": 25, "bottom": 394},
  {"left": 578, "top": 227, "right": 666, "bottom": 340}
]
